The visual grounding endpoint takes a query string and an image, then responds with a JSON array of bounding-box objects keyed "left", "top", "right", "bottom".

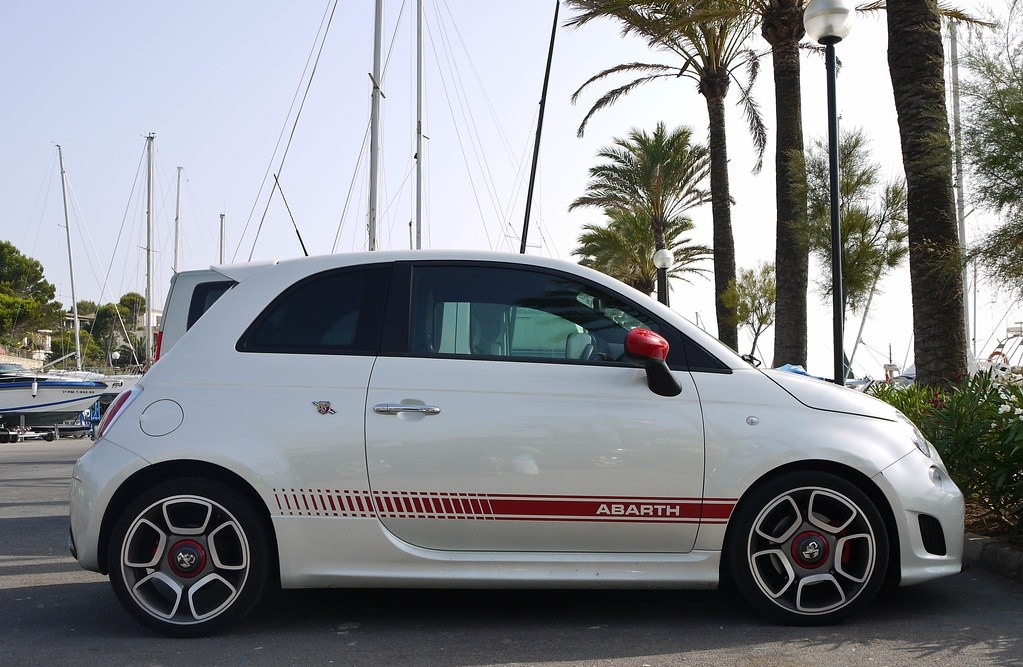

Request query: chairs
[{"left": 469, "top": 312, "right": 504, "bottom": 355}]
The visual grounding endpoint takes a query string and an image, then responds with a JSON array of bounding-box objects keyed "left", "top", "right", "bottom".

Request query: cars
[{"left": 66, "top": 255, "right": 967, "bottom": 625}]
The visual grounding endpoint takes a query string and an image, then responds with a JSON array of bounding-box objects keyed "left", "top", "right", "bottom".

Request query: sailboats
[{"left": 5, "top": 141, "right": 143, "bottom": 414}]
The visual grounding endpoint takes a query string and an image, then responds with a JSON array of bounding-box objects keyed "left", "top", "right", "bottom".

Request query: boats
[{"left": 0, "top": 363, "right": 107, "bottom": 428}]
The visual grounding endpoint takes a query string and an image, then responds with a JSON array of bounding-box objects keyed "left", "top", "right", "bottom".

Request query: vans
[{"left": 152, "top": 263, "right": 589, "bottom": 359}]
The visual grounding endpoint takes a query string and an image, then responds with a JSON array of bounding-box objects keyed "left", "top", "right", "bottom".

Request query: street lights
[
  {"left": 802, "top": 0, "right": 855, "bottom": 385},
  {"left": 111, "top": 351, "right": 121, "bottom": 373},
  {"left": 653, "top": 249, "right": 675, "bottom": 307}
]
